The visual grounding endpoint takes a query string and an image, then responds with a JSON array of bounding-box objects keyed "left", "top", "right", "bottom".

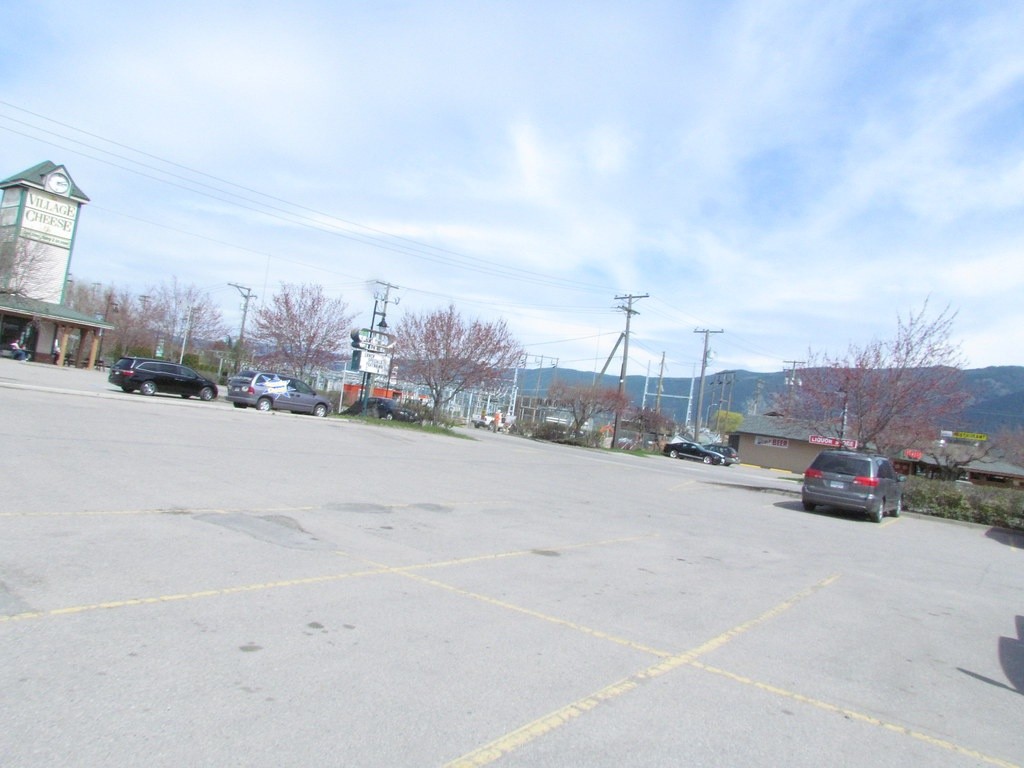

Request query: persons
[
  {"left": 502, "top": 412, "right": 511, "bottom": 434},
  {"left": 9, "top": 338, "right": 32, "bottom": 363},
  {"left": 493, "top": 409, "right": 501, "bottom": 435},
  {"left": 52, "top": 338, "right": 61, "bottom": 365}
]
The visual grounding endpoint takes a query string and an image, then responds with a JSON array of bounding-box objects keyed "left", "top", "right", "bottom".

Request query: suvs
[{"left": 702, "top": 442, "right": 738, "bottom": 466}]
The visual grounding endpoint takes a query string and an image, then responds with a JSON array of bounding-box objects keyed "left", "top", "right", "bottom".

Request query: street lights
[
  {"left": 97, "top": 302, "right": 119, "bottom": 361},
  {"left": 358, "top": 299, "right": 389, "bottom": 402}
]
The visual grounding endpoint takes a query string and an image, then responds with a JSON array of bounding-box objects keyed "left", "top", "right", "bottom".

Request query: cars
[
  {"left": 369, "top": 396, "right": 418, "bottom": 423},
  {"left": 663, "top": 442, "right": 725, "bottom": 465}
]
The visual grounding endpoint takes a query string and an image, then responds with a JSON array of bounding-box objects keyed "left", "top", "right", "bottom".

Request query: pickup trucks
[{"left": 472, "top": 412, "right": 506, "bottom": 432}]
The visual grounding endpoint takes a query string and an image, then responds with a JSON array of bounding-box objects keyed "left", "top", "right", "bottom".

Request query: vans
[
  {"left": 108, "top": 357, "right": 218, "bottom": 400},
  {"left": 225, "top": 370, "right": 331, "bottom": 417},
  {"left": 802, "top": 449, "right": 906, "bottom": 523}
]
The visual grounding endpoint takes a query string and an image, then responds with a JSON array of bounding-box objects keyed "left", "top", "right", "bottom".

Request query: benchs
[{"left": 64, "top": 353, "right": 105, "bottom": 370}]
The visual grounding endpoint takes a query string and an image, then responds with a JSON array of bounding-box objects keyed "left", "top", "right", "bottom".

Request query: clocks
[{"left": 49, "top": 173, "right": 71, "bottom": 194}]
